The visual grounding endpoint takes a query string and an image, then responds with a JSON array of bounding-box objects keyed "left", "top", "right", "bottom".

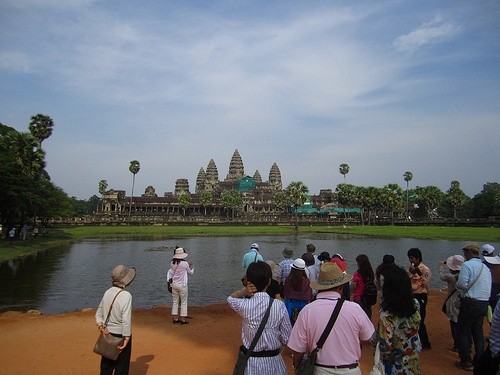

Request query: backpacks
[{"left": 360, "top": 272, "right": 377, "bottom": 305}]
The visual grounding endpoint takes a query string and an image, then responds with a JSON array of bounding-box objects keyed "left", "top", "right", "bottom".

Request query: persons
[
  {"left": 9, "top": 227, "right": 16, "bottom": 247},
  {"left": 376, "top": 254, "right": 400, "bottom": 291},
  {"left": 33, "top": 225, "right": 39, "bottom": 241},
  {"left": 287, "top": 261, "right": 376, "bottom": 375},
  {"left": 242, "top": 243, "right": 263, "bottom": 298},
  {"left": 407, "top": 247, "right": 433, "bottom": 350},
  {"left": 95, "top": 265, "right": 137, "bottom": 375},
  {"left": 166, "top": 246, "right": 195, "bottom": 324},
  {"left": 43, "top": 225, "right": 47, "bottom": 238},
  {"left": 227, "top": 262, "right": 293, "bottom": 375},
  {"left": 455, "top": 243, "right": 496, "bottom": 370},
  {"left": 377, "top": 268, "right": 424, "bottom": 375},
  {"left": 266, "top": 243, "right": 375, "bottom": 343},
  {"left": 22, "top": 224, "right": 27, "bottom": 240},
  {"left": 473, "top": 256, "right": 500, "bottom": 375},
  {"left": 438, "top": 255, "right": 472, "bottom": 352}
]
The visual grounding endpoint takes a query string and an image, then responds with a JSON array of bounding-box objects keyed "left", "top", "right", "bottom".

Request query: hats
[
  {"left": 265, "top": 260, "right": 279, "bottom": 276},
  {"left": 111, "top": 265, "right": 136, "bottom": 288},
  {"left": 483, "top": 253, "right": 500, "bottom": 264},
  {"left": 309, "top": 262, "right": 353, "bottom": 290},
  {"left": 282, "top": 247, "right": 293, "bottom": 257},
  {"left": 330, "top": 258, "right": 347, "bottom": 272},
  {"left": 251, "top": 243, "right": 259, "bottom": 250},
  {"left": 290, "top": 258, "right": 306, "bottom": 270},
  {"left": 301, "top": 253, "right": 315, "bottom": 266},
  {"left": 463, "top": 242, "right": 480, "bottom": 254},
  {"left": 173, "top": 248, "right": 188, "bottom": 259},
  {"left": 447, "top": 254, "right": 465, "bottom": 270},
  {"left": 480, "top": 244, "right": 495, "bottom": 255}
]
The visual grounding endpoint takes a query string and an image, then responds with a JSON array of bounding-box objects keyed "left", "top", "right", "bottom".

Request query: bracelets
[
  {"left": 123, "top": 338, "right": 130, "bottom": 343},
  {"left": 98, "top": 323, "right": 105, "bottom": 329}
]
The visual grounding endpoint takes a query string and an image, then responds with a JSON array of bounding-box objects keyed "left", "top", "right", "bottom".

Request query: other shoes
[
  {"left": 173, "top": 318, "right": 181, "bottom": 323},
  {"left": 181, "top": 320, "right": 189, "bottom": 324},
  {"left": 451, "top": 347, "right": 458, "bottom": 353},
  {"left": 455, "top": 360, "right": 473, "bottom": 370}
]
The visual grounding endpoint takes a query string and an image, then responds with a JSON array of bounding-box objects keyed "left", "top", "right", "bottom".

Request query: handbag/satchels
[
  {"left": 488, "top": 305, "right": 493, "bottom": 323},
  {"left": 167, "top": 278, "right": 172, "bottom": 293},
  {"left": 296, "top": 349, "right": 318, "bottom": 375},
  {"left": 442, "top": 301, "right": 447, "bottom": 315},
  {"left": 369, "top": 342, "right": 386, "bottom": 375},
  {"left": 232, "top": 350, "right": 247, "bottom": 375},
  {"left": 93, "top": 331, "right": 124, "bottom": 360}
]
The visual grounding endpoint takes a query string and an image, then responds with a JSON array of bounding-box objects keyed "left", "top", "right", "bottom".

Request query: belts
[
  {"left": 249, "top": 348, "right": 280, "bottom": 357},
  {"left": 316, "top": 363, "right": 358, "bottom": 369}
]
